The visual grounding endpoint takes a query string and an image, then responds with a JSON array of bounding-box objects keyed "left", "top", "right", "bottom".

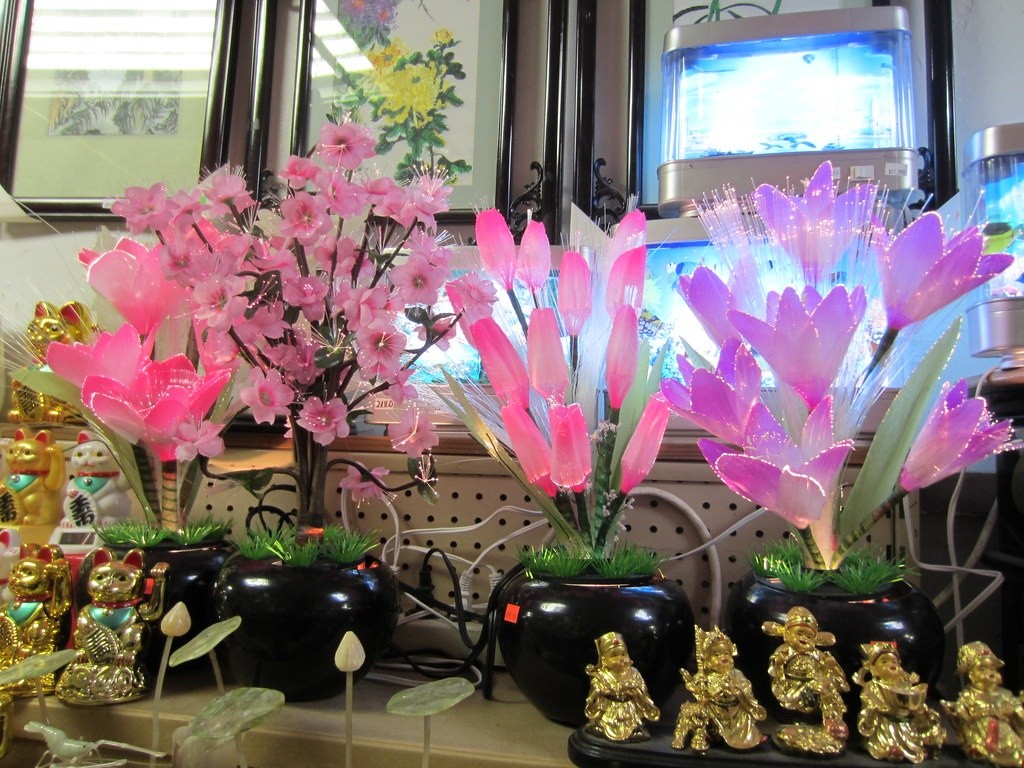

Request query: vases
[
  {"left": 721, "top": 571, "right": 954, "bottom": 705},
  {"left": 74, "top": 528, "right": 242, "bottom": 687},
  {"left": 492, "top": 570, "right": 697, "bottom": 730},
  {"left": 216, "top": 554, "right": 401, "bottom": 701}
]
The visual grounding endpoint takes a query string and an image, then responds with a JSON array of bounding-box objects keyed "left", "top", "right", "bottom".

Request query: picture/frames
[
  {"left": 290, "top": 0, "right": 516, "bottom": 222},
  {"left": 0, "top": 0, "right": 242, "bottom": 222}
]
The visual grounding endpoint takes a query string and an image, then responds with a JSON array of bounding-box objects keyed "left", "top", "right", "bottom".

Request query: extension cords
[{"left": 391, "top": 613, "right": 506, "bottom": 667}]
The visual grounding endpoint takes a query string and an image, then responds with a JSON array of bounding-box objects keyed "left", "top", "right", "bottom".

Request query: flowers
[
  {"left": 115, "top": 119, "right": 496, "bottom": 566},
  {"left": 433, "top": 213, "right": 670, "bottom": 580},
  {"left": 658, "top": 155, "right": 1024, "bottom": 592},
  {"left": 5, "top": 238, "right": 241, "bottom": 543}
]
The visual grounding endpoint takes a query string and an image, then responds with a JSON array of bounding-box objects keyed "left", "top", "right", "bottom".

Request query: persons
[
  {"left": 762, "top": 606, "right": 851, "bottom": 740},
  {"left": 851, "top": 640, "right": 947, "bottom": 763},
  {"left": 671, "top": 624, "right": 768, "bottom": 751},
  {"left": 584, "top": 632, "right": 661, "bottom": 744},
  {"left": 939, "top": 640, "right": 1024, "bottom": 768}
]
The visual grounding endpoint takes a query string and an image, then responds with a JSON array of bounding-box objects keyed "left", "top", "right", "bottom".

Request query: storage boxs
[
  {"left": 961, "top": 122, "right": 1023, "bottom": 357},
  {"left": 658, "top": 6, "right": 915, "bottom": 219}
]
